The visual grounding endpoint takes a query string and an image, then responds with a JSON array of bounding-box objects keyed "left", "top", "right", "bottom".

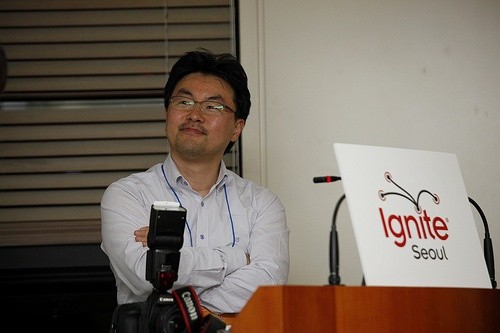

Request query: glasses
[{"left": 167, "top": 96, "right": 237, "bottom": 118}]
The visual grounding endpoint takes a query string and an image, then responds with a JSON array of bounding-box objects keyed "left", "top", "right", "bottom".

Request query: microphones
[{"left": 313, "top": 176, "right": 340, "bottom": 183}]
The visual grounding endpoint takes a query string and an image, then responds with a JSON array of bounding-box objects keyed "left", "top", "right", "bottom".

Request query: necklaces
[{"left": 161, "top": 165, "right": 235, "bottom": 249}]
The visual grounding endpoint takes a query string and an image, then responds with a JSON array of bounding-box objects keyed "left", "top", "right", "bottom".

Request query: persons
[{"left": 99, "top": 45, "right": 291, "bottom": 313}]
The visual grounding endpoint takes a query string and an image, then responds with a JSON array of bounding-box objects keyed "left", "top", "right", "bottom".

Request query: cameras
[{"left": 108, "top": 201, "right": 217, "bottom": 333}]
